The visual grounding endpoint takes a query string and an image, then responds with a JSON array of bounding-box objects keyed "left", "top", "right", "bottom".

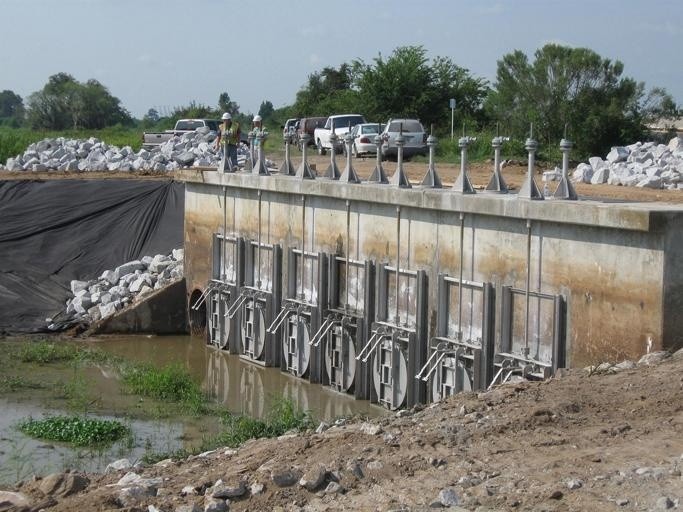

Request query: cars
[
  {"left": 280, "top": 117, "right": 328, "bottom": 151},
  {"left": 343, "top": 123, "right": 386, "bottom": 158},
  {"left": 381, "top": 117, "right": 428, "bottom": 159}
]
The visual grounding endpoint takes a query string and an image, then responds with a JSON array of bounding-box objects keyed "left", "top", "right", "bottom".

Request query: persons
[
  {"left": 215, "top": 113, "right": 239, "bottom": 168},
  {"left": 251, "top": 115, "right": 267, "bottom": 146}
]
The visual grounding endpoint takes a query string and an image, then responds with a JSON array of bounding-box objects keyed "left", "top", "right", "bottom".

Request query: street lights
[{"left": 449, "top": 99, "right": 456, "bottom": 139}]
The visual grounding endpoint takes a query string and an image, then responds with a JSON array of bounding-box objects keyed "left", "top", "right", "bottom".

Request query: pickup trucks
[
  {"left": 141, "top": 117, "right": 250, "bottom": 153},
  {"left": 313, "top": 114, "right": 367, "bottom": 155}
]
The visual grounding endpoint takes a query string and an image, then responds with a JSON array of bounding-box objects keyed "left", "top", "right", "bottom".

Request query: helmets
[
  {"left": 252, "top": 115, "right": 262, "bottom": 121},
  {"left": 221, "top": 112, "right": 232, "bottom": 119}
]
